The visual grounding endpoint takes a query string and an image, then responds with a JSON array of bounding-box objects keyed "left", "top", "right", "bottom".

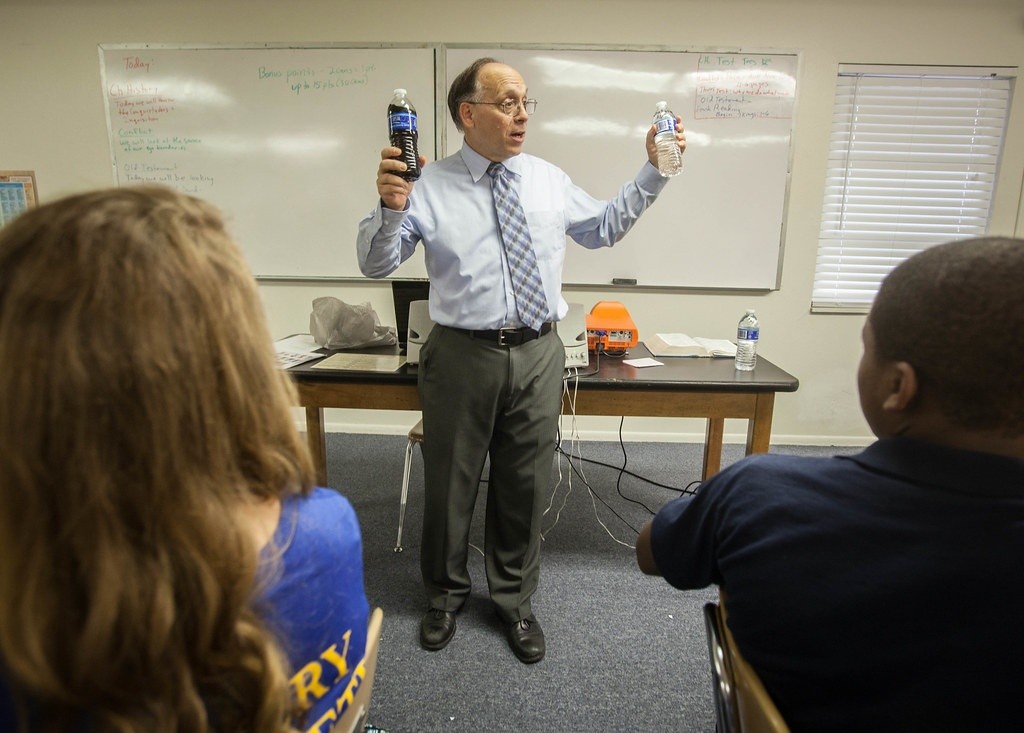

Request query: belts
[{"left": 474, "top": 322, "right": 552, "bottom": 347}]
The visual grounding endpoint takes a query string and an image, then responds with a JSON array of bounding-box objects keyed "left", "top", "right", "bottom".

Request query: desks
[{"left": 272, "top": 332, "right": 801, "bottom": 489}]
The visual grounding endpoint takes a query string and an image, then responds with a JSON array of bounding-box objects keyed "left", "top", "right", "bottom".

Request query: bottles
[
  {"left": 734, "top": 309, "right": 760, "bottom": 371},
  {"left": 388, "top": 87, "right": 421, "bottom": 184},
  {"left": 653, "top": 99, "right": 682, "bottom": 178}
]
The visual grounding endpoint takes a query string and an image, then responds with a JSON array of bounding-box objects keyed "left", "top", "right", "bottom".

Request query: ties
[{"left": 486, "top": 162, "right": 550, "bottom": 331}]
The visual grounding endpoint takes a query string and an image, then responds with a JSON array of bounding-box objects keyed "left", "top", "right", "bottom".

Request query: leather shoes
[
  {"left": 420, "top": 597, "right": 465, "bottom": 651},
  {"left": 493, "top": 608, "right": 546, "bottom": 663}
]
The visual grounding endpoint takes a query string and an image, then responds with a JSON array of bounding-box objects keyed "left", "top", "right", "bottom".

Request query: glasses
[{"left": 464, "top": 99, "right": 539, "bottom": 116}]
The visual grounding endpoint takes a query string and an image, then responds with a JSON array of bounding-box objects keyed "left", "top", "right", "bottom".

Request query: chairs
[
  {"left": 702, "top": 582, "right": 790, "bottom": 732},
  {"left": 393, "top": 417, "right": 427, "bottom": 553}
]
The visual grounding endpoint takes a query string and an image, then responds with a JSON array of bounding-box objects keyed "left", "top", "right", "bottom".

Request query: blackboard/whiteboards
[
  {"left": 98, "top": 41, "right": 436, "bottom": 282},
  {"left": 437, "top": 42, "right": 799, "bottom": 291}
]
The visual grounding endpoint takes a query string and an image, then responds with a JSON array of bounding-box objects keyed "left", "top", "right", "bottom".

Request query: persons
[
  {"left": 633, "top": 233, "right": 1024, "bottom": 733},
  {"left": 0, "top": 177, "right": 375, "bottom": 733},
  {"left": 354, "top": 53, "right": 688, "bottom": 665}
]
